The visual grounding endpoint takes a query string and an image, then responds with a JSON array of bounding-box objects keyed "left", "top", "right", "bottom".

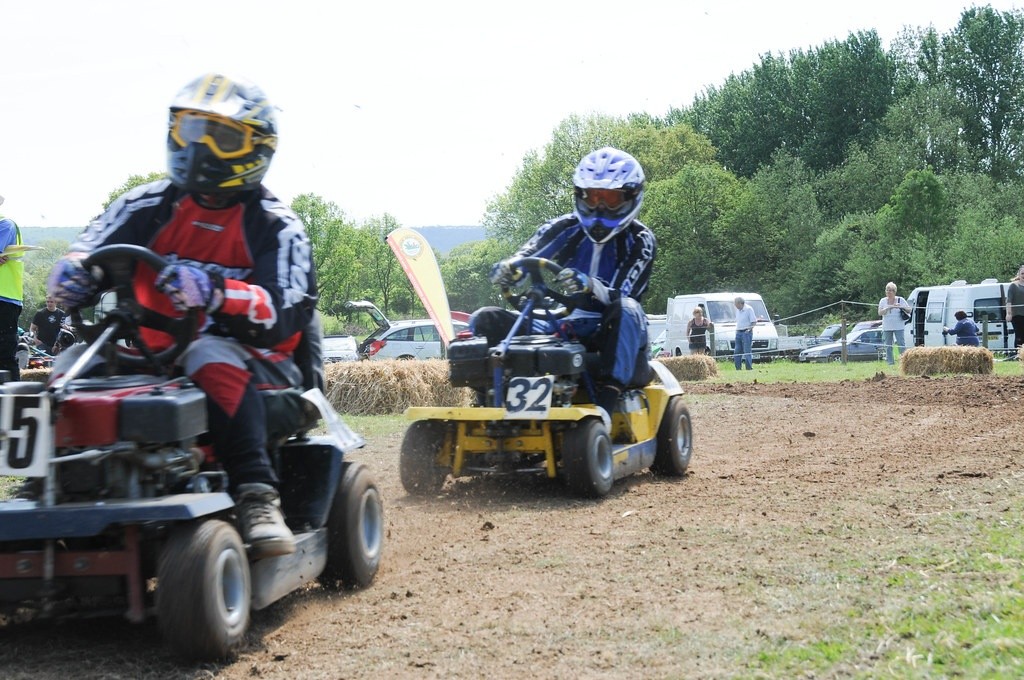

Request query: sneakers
[{"left": 233, "top": 483, "right": 296, "bottom": 557}]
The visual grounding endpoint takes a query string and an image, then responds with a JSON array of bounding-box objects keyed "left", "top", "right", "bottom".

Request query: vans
[
  {"left": 906, "top": 278, "right": 1015, "bottom": 349},
  {"left": 665, "top": 292, "right": 779, "bottom": 357}
]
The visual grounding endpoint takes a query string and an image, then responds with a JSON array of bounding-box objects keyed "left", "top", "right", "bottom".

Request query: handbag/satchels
[{"left": 897, "top": 297, "right": 911, "bottom": 320}]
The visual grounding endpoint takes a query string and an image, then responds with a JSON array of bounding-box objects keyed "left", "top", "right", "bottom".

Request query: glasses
[
  {"left": 171, "top": 110, "right": 254, "bottom": 160},
  {"left": 579, "top": 186, "right": 630, "bottom": 211}
]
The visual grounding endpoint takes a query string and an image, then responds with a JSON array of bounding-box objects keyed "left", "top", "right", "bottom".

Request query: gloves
[
  {"left": 553, "top": 268, "right": 594, "bottom": 298},
  {"left": 154, "top": 264, "right": 212, "bottom": 312},
  {"left": 46, "top": 250, "right": 101, "bottom": 306},
  {"left": 488, "top": 259, "right": 515, "bottom": 286}
]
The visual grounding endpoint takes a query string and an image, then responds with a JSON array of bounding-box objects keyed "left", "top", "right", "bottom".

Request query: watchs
[{"left": 747, "top": 329, "right": 751, "bottom": 332}]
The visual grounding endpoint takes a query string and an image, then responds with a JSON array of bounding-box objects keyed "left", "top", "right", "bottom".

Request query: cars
[
  {"left": 852, "top": 320, "right": 882, "bottom": 339},
  {"left": 819, "top": 324, "right": 849, "bottom": 343},
  {"left": 798, "top": 328, "right": 886, "bottom": 364},
  {"left": 647, "top": 315, "right": 666, "bottom": 357}
]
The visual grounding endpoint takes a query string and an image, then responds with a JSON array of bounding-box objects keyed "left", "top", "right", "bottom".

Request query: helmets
[
  {"left": 572, "top": 147, "right": 646, "bottom": 235},
  {"left": 164, "top": 70, "right": 279, "bottom": 191}
]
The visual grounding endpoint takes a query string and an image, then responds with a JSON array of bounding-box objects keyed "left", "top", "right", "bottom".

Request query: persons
[
  {"left": 29, "top": 293, "right": 66, "bottom": 356},
  {"left": 43, "top": 72, "right": 323, "bottom": 560},
  {"left": 466, "top": 145, "right": 656, "bottom": 439},
  {"left": 0, "top": 192, "right": 24, "bottom": 382},
  {"left": 1005, "top": 264, "right": 1024, "bottom": 361},
  {"left": 687, "top": 306, "right": 714, "bottom": 356},
  {"left": 943, "top": 311, "right": 980, "bottom": 347},
  {"left": 878, "top": 282, "right": 912, "bottom": 365},
  {"left": 734, "top": 296, "right": 757, "bottom": 370}
]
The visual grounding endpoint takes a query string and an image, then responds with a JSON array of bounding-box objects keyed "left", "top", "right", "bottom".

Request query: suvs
[{"left": 343, "top": 299, "right": 472, "bottom": 361}]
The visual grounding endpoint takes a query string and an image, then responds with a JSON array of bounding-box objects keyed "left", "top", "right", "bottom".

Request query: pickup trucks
[{"left": 325, "top": 335, "right": 359, "bottom": 362}]
